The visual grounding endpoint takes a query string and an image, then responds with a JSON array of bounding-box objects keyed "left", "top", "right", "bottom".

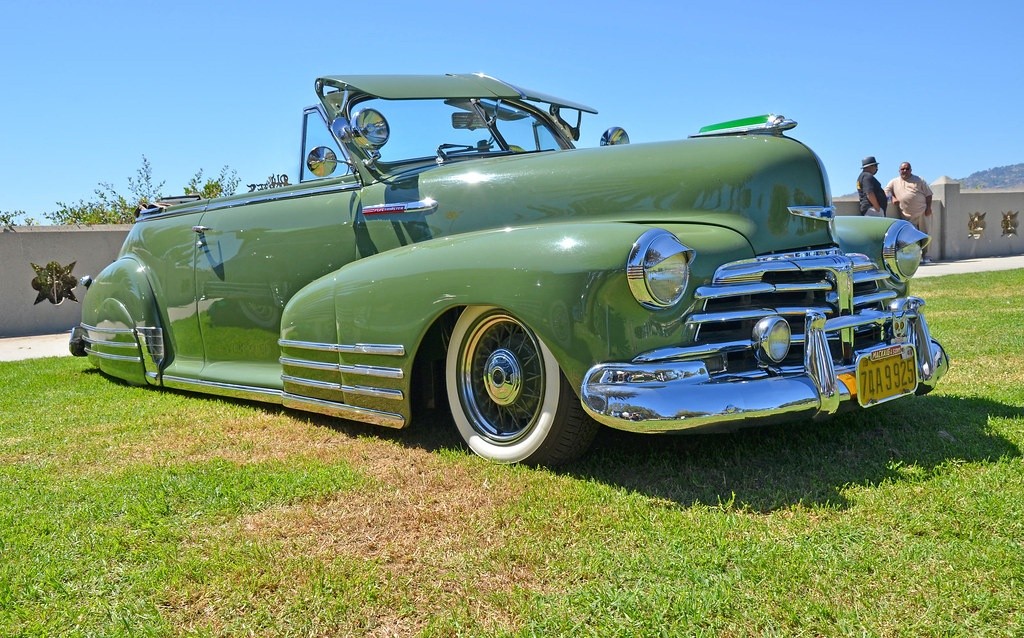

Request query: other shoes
[{"left": 920, "top": 256, "right": 935, "bottom": 265}]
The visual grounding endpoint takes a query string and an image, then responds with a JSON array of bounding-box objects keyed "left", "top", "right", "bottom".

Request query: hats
[{"left": 860, "top": 156, "right": 879, "bottom": 169}]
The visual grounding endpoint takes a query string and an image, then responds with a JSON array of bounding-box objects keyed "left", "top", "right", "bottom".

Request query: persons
[
  {"left": 883, "top": 162, "right": 934, "bottom": 262},
  {"left": 856, "top": 157, "right": 888, "bottom": 218}
]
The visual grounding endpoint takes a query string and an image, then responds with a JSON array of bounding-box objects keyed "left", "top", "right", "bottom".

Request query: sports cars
[{"left": 70, "top": 71, "right": 949, "bottom": 472}]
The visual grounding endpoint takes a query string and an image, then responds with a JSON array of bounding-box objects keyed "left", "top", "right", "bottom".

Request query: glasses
[{"left": 900, "top": 168, "right": 911, "bottom": 171}]
